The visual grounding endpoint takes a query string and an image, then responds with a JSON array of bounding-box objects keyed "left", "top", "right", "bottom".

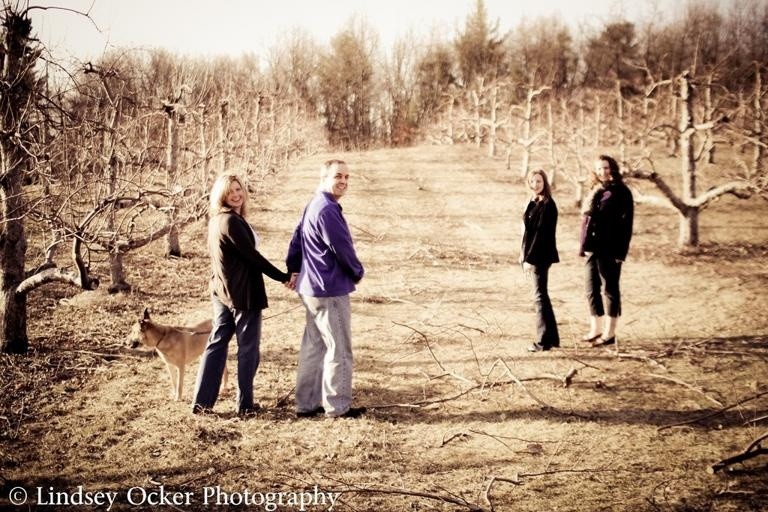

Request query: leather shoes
[
  {"left": 235, "top": 402, "right": 259, "bottom": 420},
  {"left": 191, "top": 406, "right": 220, "bottom": 419},
  {"left": 297, "top": 406, "right": 325, "bottom": 419},
  {"left": 333, "top": 406, "right": 367, "bottom": 419}
]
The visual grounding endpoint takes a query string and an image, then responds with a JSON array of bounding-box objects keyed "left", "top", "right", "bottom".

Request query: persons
[
  {"left": 187, "top": 172, "right": 298, "bottom": 421},
  {"left": 286, "top": 158, "right": 369, "bottom": 418},
  {"left": 516, "top": 167, "right": 562, "bottom": 351},
  {"left": 577, "top": 153, "right": 637, "bottom": 347}
]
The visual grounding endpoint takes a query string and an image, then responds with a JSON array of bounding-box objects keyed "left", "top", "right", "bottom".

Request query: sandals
[
  {"left": 580, "top": 330, "right": 616, "bottom": 348},
  {"left": 527, "top": 339, "right": 561, "bottom": 353}
]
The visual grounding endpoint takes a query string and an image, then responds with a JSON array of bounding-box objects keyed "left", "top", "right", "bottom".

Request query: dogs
[{"left": 125, "top": 308, "right": 229, "bottom": 401}]
